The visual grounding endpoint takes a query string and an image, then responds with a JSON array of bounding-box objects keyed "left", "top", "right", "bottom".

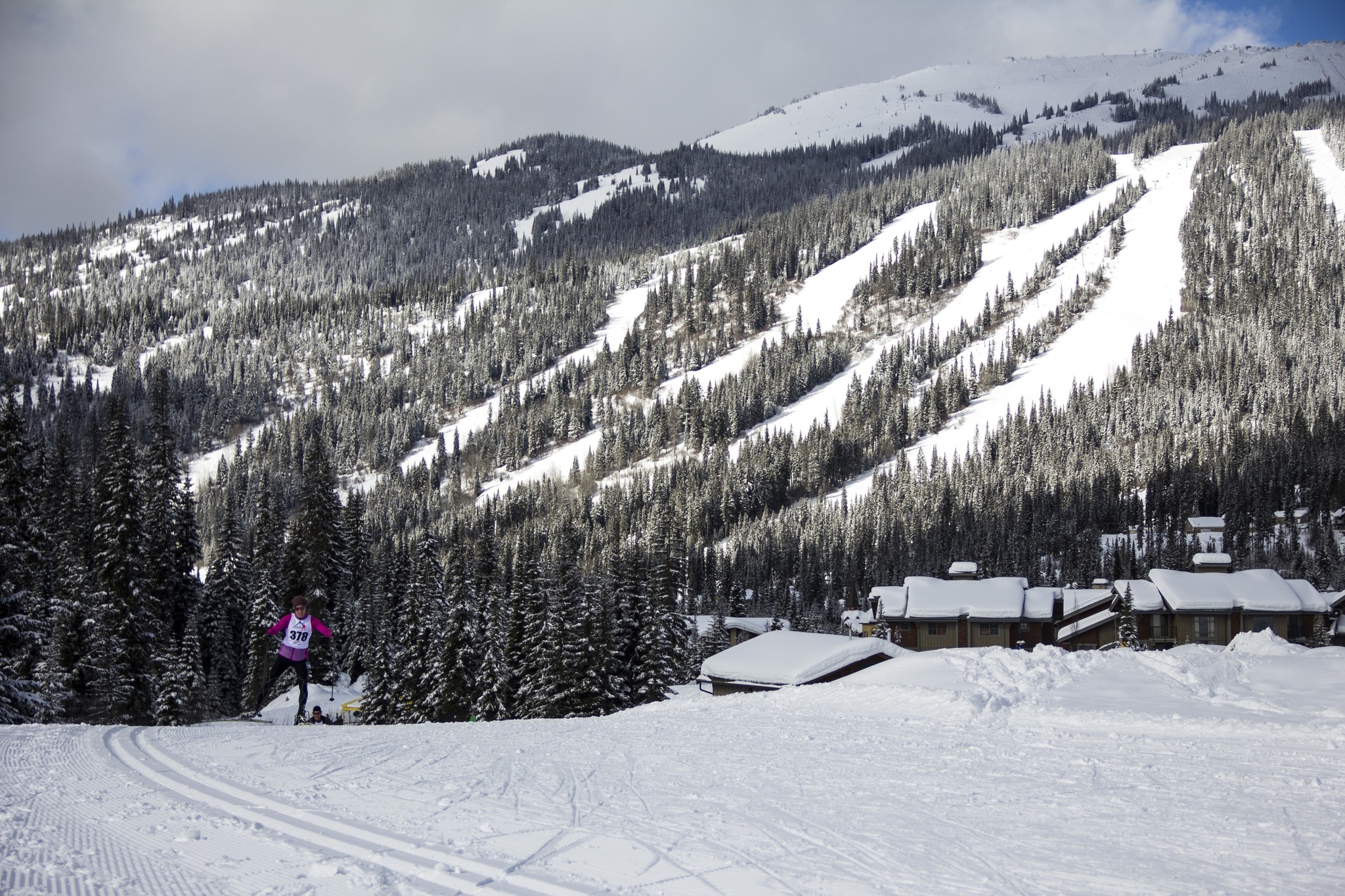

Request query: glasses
[
  {"left": 292, "top": 604, "right": 307, "bottom": 611},
  {"left": 313, "top": 712, "right": 320, "bottom": 715}
]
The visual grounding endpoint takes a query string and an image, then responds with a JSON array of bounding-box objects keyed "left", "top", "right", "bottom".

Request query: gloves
[
  {"left": 264, "top": 633, "right": 272, "bottom": 642},
  {"left": 331, "top": 632, "right": 338, "bottom": 641}
]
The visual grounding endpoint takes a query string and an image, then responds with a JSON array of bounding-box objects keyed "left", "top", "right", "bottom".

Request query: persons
[
  {"left": 241, "top": 596, "right": 340, "bottom": 725},
  {"left": 309, "top": 706, "right": 334, "bottom": 726}
]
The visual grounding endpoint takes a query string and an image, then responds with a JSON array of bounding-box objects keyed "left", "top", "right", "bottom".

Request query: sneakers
[
  {"left": 241, "top": 708, "right": 259, "bottom": 719},
  {"left": 298, "top": 710, "right": 312, "bottom": 724}
]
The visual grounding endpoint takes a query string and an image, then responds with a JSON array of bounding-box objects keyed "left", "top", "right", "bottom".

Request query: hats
[
  {"left": 313, "top": 706, "right": 321, "bottom": 712},
  {"left": 292, "top": 595, "right": 308, "bottom": 609}
]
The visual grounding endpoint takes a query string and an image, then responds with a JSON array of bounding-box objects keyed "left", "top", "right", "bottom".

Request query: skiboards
[{"left": 219, "top": 715, "right": 317, "bottom": 726}]
